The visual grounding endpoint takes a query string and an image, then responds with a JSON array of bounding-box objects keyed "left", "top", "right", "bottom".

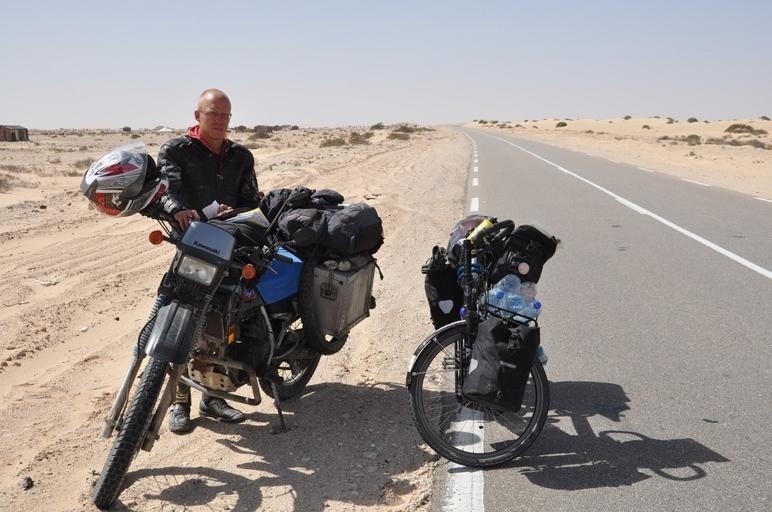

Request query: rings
[{"left": 186, "top": 214, "right": 192, "bottom": 219}]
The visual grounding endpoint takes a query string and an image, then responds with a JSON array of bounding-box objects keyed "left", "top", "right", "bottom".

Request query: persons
[{"left": 157, "top": 89, "right": 268, "bottom": 435}]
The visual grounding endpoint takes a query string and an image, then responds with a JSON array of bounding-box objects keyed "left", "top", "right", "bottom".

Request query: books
[{"left": 226, "top": 207, "right": 270, "bottom": 229}]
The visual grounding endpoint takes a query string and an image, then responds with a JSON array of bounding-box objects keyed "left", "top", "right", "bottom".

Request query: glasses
[{"left": 199, "top": 110, "right": 231, "bottom": 121}]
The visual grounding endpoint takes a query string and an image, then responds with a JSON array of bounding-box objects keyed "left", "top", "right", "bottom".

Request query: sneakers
[
  {"left": 168, "top": 403, "right": 191, "bottom": 432},
  {"left": 199, "top": 398, "right": 244, "bottom": 423}
]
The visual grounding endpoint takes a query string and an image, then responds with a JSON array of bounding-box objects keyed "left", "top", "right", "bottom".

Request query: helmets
[{"left": 80, "top": 142, "right": 170, "bottom": 218}]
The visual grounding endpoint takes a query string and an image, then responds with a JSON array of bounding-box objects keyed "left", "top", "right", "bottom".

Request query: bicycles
[{"left": 405, "top": 218, "right": 554, "bottom": 467}]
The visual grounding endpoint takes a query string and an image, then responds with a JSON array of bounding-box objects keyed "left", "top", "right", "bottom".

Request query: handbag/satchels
[
  {"left": 489, "top": 224, "right": 556, "bottom": 283},
  {"left": 278, "top": 204, "right": 382, "bottom": 256},
  {"left": 425, "top": 246, "right": 462, "bottom": 330},
  {"left": 462, "top": 317, "right": 540, "bottom": 412}
]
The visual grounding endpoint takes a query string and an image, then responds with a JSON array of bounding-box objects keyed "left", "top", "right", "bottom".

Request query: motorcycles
[{"left": 88, "top": 143, "right": 378, "bottom": 507}]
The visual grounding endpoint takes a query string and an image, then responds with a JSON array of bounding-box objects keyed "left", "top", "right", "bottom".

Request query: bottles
[
  {"left": 536, "top": 344, "right": 548, "bottom": 366},
  {"left": 456, "top": 274, "right": 542, "bottom": 326}
]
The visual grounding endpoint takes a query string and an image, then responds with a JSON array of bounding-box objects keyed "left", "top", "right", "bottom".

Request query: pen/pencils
[{"left": 188, "top": 216, "right": 201, "bottom": 219}]
